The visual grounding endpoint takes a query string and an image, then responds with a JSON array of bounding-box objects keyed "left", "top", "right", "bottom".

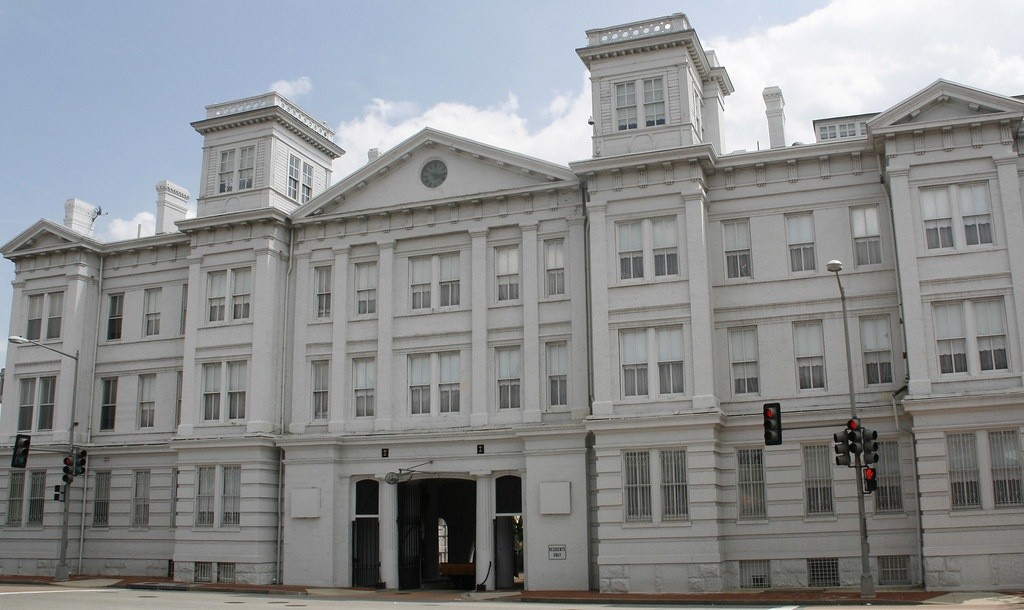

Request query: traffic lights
[
  {"left": 10, "top": 434, "right": 31, "bottom": 469},
  {"left": 847, "top": 417, "right": 864, "bottom": 456},
  {"left": 73, "top": 447, "right": 87, "bottom": 476},
  {"left": 54, "top": 484, "right": 65, "bottom": 501},
  {"left": 862, "top": 427, "right": 881, "bottom": 465},
  {"left": 762, "top": 402, "right": 783, "bottom": 446},
  {"left": 834, "top": 430, "right": 853, "bottom": 466},
  {"left": 60, "top": 453, "right": 74, "bottom": 484},
  {"left": 864, "top": 466, "right": 878, "bottom": 491}
]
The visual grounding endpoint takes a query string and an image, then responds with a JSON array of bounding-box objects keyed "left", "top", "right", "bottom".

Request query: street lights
[
  {"left": 826, "top": 260, "right": 878, "bottom": 600},
  {"left": 8, "top": 335, "right": 80, "bottom": 583}
]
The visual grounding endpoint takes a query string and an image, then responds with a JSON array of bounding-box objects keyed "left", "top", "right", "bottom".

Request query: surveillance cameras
[{"left": 588, "top": 119, "right": 595, "bottom": 125}]
